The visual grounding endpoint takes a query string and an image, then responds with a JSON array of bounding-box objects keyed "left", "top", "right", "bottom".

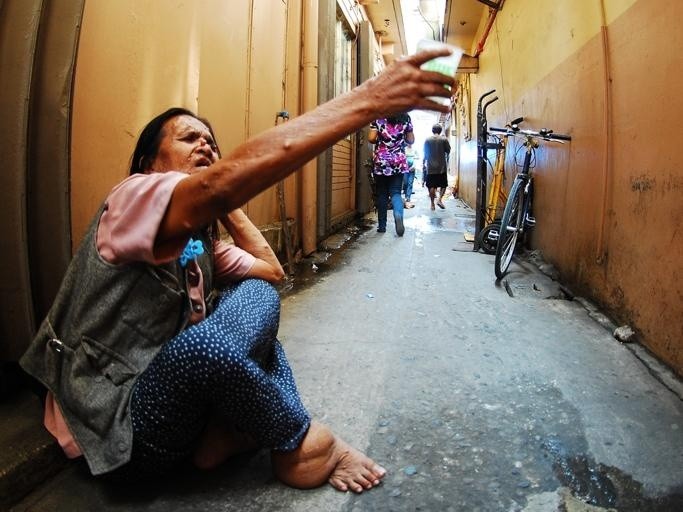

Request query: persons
[
  {"left": 367, "top": 112, "right": 414, "bottom": 237},
  {"left": 18, "top": 45, "right": 458, "bottom": 496},
  {"left": 422, "top": 123, "right": 452, "bottom": 210},
  {"left": 401, "top": 160, "right": 415, "bottom": 202}
]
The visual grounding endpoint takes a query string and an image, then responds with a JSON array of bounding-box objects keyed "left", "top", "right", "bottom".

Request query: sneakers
[
  {"left": 377, "top": 228, "right": 385, "bottom": 232},
  {"left": 395, "top": 215, "right": 404, "bottom": 236},
  {"left": 430, "top": 202, "right": 445, "bottom": 210}
]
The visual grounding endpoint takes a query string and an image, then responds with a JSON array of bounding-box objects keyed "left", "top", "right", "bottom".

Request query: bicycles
[{"left": 484, "top": 123, "right": 572, "bottom": 282}]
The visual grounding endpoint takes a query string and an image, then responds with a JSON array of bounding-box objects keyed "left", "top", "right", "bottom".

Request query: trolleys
[
  {"left": 449, "top": 87, "right": 502, "bottom": 254},
  {"left": 463, "top": 116, "right": 524, "bottom": 243}
]
